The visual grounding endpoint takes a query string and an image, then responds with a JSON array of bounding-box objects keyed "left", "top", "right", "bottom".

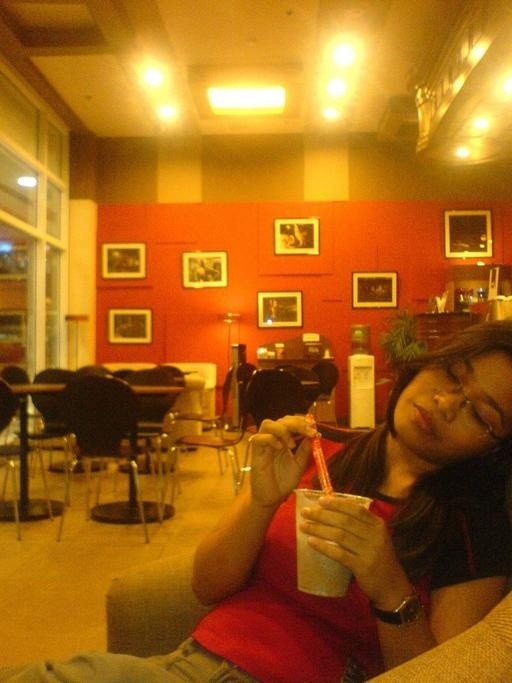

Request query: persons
[
  {"left": 365, "top": 279, "right": 390, "bottom": 301},
  {"left": 199, "top": 259, "right": 220, "bottom": 282},
  {"left": 1, "top": 319, "right": 512, "bottom": 683}
]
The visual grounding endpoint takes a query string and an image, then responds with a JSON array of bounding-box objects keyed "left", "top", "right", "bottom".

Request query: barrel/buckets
[{"left": 351, "top": 325, "right": 371, "bottom": 356}]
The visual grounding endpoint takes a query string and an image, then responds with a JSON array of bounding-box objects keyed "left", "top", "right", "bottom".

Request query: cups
[{"left": 293, "top": 487, "right": 374, "bottom": 599}]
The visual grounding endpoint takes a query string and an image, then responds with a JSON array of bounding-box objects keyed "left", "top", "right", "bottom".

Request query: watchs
[{"left": 373, "top": 587, "right": 424, "bottom": 627}]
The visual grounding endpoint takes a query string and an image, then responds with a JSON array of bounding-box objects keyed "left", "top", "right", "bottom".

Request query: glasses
[{"left": 430, "top": 354, "right": 505, "bottom": 447}]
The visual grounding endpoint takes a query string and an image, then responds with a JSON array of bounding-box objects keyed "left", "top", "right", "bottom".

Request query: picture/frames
[
  {"left": 102, "top": 241, "right": 146, "bottom": 280},
  {"left": 182, "top": 252, "right": 228, "bottom": 288},
  {"left": 110, "top": 308, "right": 152, "bottom": 344},
  {"left": 443, "top": 209, "right": 493, "bottom": 258},
  {"left": 351, "top": 273, "right": 398, "bottom": 307},
  {"left": 276, "top": 218, "right": 319, "bottom": 254},
  {"left": 258, "top": 290, "right": 302, "bottom": 328}
]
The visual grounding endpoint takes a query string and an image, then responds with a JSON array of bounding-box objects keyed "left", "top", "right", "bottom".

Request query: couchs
[{"left": 102, "top": 541, "right": 511, "bottom": 682}]
